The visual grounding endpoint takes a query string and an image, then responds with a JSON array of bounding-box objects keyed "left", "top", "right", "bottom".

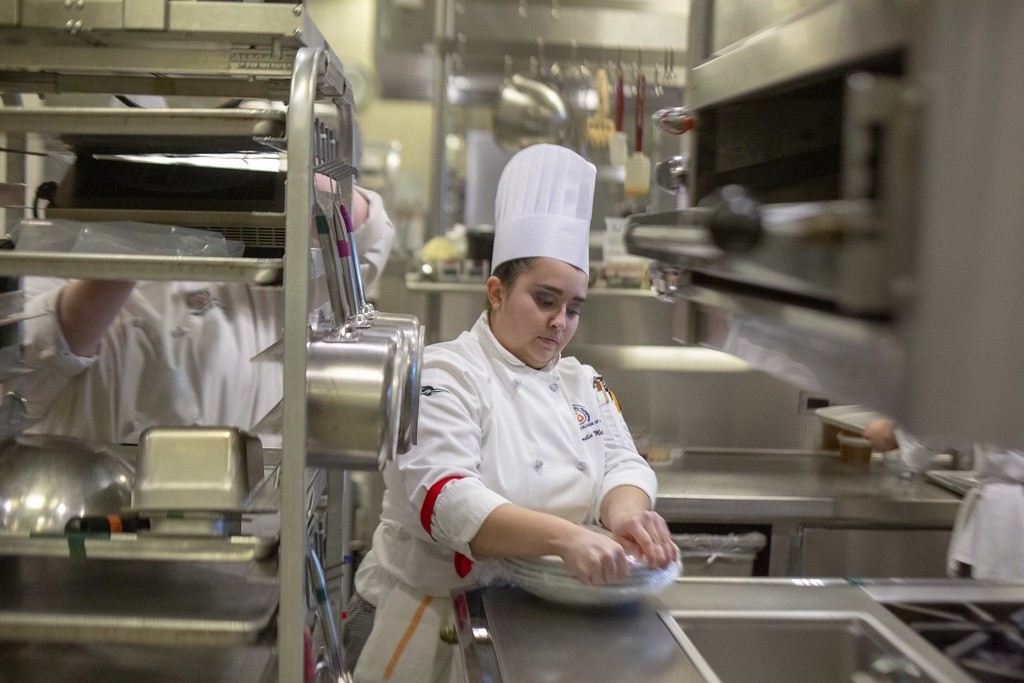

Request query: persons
[
  {"left": 10, "top": 170, "right": 394, "bottom": 448},
  {"left": 351, "top": 143, "right": 679, "bottom": 683}
]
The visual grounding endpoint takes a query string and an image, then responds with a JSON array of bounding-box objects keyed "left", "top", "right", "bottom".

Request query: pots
[{"left": 305, "top": 172, "right": 424, "bottom": 473}]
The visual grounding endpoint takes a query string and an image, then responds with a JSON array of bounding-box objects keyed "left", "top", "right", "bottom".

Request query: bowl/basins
[
  {"left": 0, "top": 437, "right": 139, "bottom": 536},
  {"left": 494, "top": 74, "right": 571, "bottom": 156}
]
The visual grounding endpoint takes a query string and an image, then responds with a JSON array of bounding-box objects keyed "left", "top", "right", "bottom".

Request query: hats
[{"left": 489, "top": 144, "right": 596, "bottom": 277}]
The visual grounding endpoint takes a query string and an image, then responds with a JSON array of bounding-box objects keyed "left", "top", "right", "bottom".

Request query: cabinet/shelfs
[{"left": 0, "top": 0, "right": 403, "bottom": 683}]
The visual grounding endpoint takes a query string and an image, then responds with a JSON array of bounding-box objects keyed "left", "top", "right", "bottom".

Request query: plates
[{"left": 501, "top": 524, "right": 684, "bottom": 603}]
